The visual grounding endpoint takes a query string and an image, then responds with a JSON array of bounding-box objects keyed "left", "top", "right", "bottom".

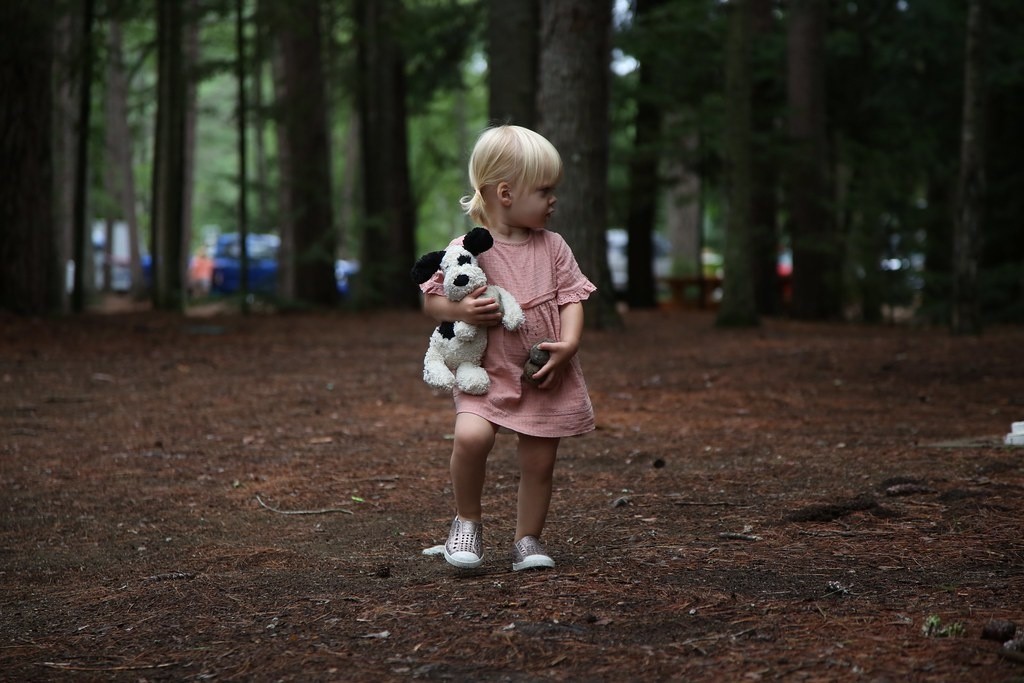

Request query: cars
[
  {"left": 606, "top": 228, "right": 672, "bottom": 284},
  {"left": 66, "top": 220, "right": 361, "bottom": 293}
]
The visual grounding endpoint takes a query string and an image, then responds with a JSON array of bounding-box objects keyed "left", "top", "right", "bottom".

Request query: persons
[{"left": 421, "top": 126, "right": 597, "bottom": 572}]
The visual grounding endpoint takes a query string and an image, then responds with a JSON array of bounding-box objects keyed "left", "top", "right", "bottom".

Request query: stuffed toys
[{"left": 413, "top": 227, "right": 526, "bottom": 395}]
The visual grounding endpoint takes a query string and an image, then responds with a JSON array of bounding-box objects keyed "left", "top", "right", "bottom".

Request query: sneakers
[
  {"left": 512, "top": 535, "right": 555, "bottom": 572},
  {"left": 444, "top": 515, "right": 486, "bottom": 568}
]
